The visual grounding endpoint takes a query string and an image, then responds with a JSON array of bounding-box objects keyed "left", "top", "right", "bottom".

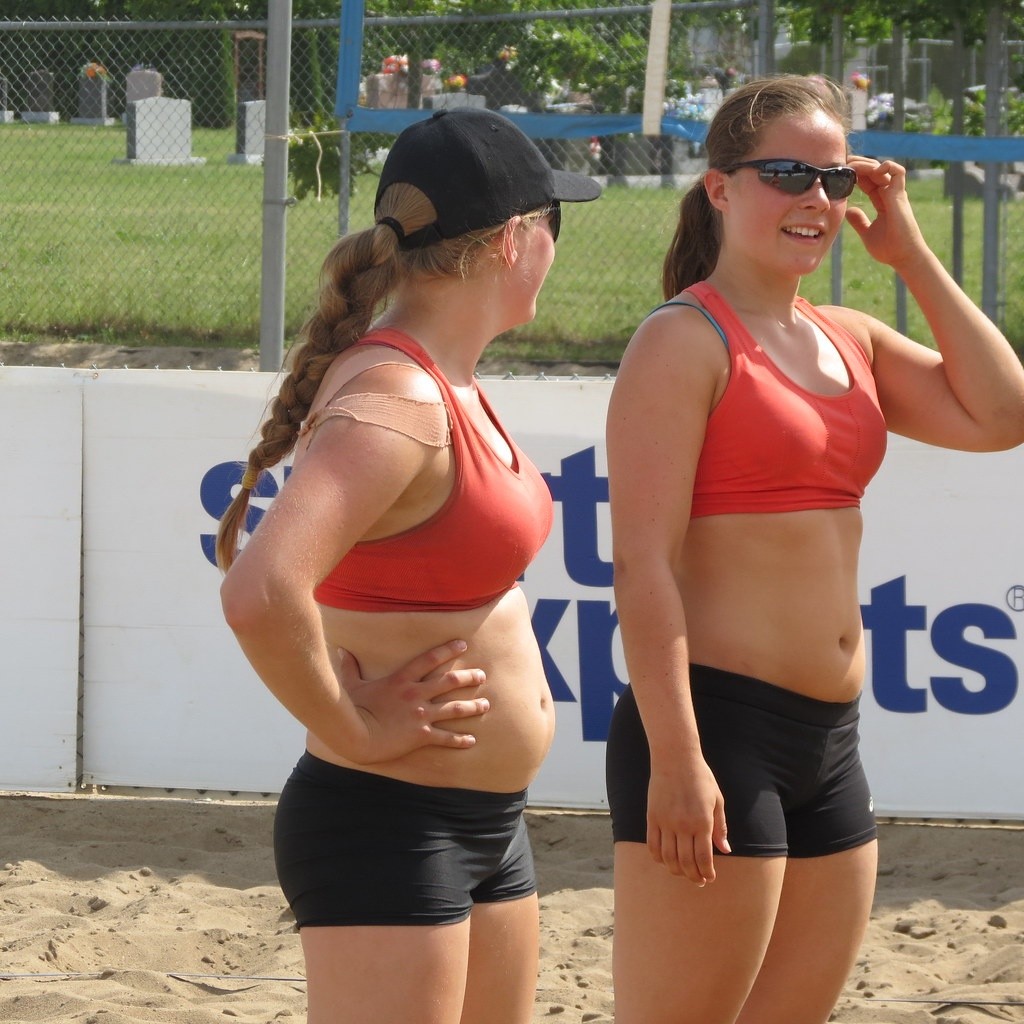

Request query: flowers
[
  {"left": 81, "top": 62, "right": 110, "bottom": 81},
  {"left": 421, "top": 59, "right": 440, "bottom": 75},
  {"left": 852, "top": 73, "right": 872, "bottom": 91},
  {"left": 497, "top": 46, "right": 518, "bottom": 69},
  {"left": 383, "top": 54, "right": 407, "bottom": 75},
  {"left": 444, "top": 75, "right": 466, "bottom": 92}
]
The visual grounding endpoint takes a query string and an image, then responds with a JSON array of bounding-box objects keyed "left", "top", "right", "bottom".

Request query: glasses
[
  {"left": 719, "top": 158, "right": 859, "bottom": 200},
  {"left": 524, "top": 200, "right": 562, "bottom": 242}
]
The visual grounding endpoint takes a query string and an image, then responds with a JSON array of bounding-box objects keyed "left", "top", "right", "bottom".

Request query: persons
[
  {"left": 606, "top": 74, "right": 1024, "bottom": 1024},
  {"left": 216, "top": 108, "right": 601, "bottom": 1023}
]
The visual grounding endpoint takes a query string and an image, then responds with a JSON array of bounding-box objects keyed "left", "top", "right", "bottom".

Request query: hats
[{"left": 373, "top": 107, "right": 603, "bottom": 250}]
